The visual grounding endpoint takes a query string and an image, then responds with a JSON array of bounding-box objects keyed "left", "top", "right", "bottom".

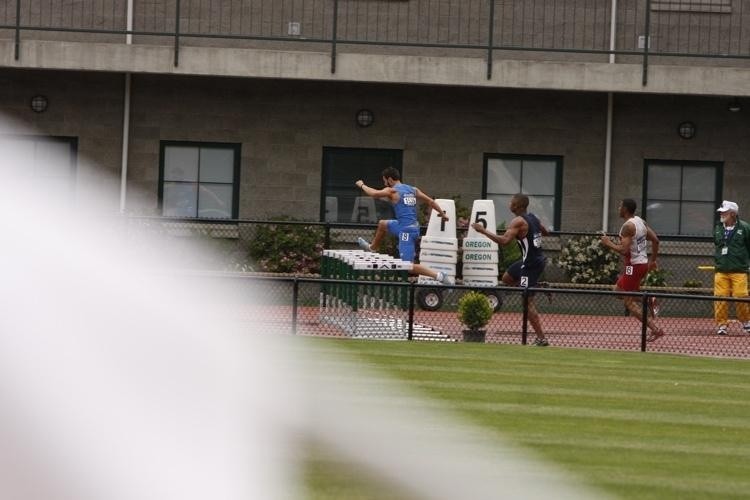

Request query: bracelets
[{"left": 359, "top": 183, "right": 364, "bottom": 190}]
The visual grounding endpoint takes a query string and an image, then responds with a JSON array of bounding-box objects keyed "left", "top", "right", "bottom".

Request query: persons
[
  {"left": 471, "top": 193, "right": 553, "bottom": 347},
  {"left": 600, "top": 198, "right": 665, "bottom": 341},
  {"left": 355, "top": 166, "right": 456, "bottom": 294},
  {"left": 713, "top": 200, "right": 750, "bottom": 336}
]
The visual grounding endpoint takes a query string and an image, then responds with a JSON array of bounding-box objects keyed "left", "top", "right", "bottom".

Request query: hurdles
[{"left": 318, "top": 248, "right": 460, "bottom": 342}]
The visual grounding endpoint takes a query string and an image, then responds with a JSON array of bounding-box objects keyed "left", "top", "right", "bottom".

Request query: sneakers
[
  {"left": 646, "top": 327, "right": 664, "bottom": 342},
  {"left": 440, "top": 272, "right": 455, "bottom": 286},
  {"left": 647, "top": 297, "right": 660, "bottom": 318},
  {"left": 358, "top": 237, "right": 370, "bottom": 252},
  {"left": 743, "top": 322, "right": 750, "bottom": 333},
  {"left": 543, "top": 282, "right": 553, "bottom": 303},
  {"left": 717, "top": 325, "right": 727, "bottom": 334},
  {"left": 531, "top": 338, "right": 548, "bottom": 346}
]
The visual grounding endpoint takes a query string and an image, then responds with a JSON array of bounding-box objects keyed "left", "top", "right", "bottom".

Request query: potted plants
[{"left": 458, "top": 292, "right": 494, "bottom": 342}]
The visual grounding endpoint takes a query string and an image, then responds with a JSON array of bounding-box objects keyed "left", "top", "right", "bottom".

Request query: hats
[{"left": 717, "top": 200, "right": 739, "bottom": 212}]
